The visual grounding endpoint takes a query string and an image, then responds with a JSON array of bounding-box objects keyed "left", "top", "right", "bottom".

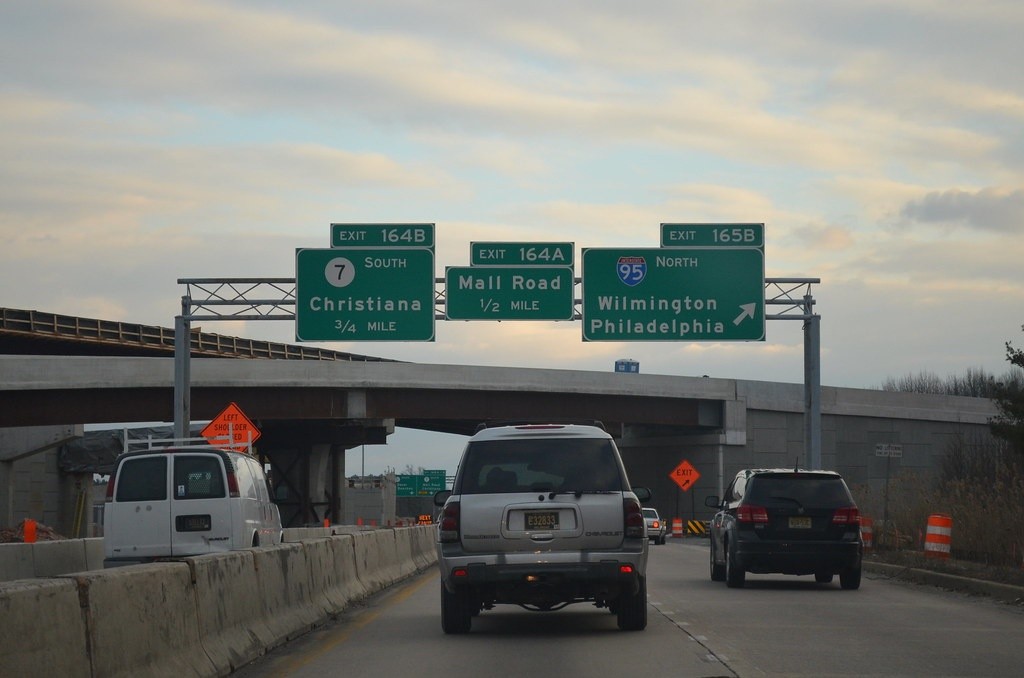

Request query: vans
[{"left": 104, "top": 446, "right": 284, "bottom": 568}]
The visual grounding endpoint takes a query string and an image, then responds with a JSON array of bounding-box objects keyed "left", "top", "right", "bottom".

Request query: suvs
[
  {"left": 710, "top": 469, "right": 864, "bottom": 590},
  {"left": 433, "top": 420, "right": 647, "bottom": 634}
]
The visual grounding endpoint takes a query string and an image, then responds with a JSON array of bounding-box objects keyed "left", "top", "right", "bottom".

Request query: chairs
[{"left": 486, "top": 470, "right": 517, "bottom": 487}]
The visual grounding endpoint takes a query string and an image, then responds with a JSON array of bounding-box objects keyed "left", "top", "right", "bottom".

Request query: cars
[{"left": 641, "top": 508, "right": 666, "bottom": 545}]
